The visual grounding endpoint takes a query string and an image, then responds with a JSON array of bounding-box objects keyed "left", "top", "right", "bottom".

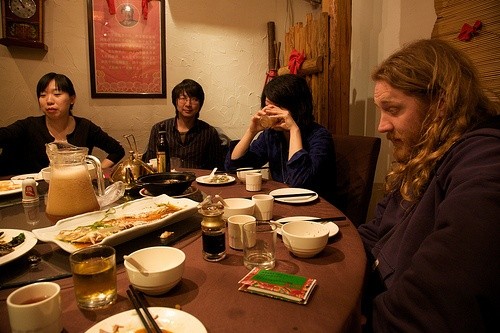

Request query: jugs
[{"left": 44, "top": 141, "right": 104, "bottom": 219}]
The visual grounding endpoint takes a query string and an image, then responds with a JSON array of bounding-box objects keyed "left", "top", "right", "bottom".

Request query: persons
[
  {"left": 142, "top": 79, "right": 223, "bottom": 172},
  {"left": 228, "top": 75, "right": 334, "bottom": 201},
  {"left": 355, "top": 39, "right": 500, "bottom": 333},
  {"left": 0, "top": 72, "right": 125, "bottom": 177}
]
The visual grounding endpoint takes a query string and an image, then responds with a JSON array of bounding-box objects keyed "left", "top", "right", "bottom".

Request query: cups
[
  {"left": 251, "top": 193, "right": 274, "bottom": 221},
  {"left": 241, "top": 221, "right": 278, "bottom": 271},
  {"left": 5, "top": 282, "right": 63, "bottom": 333},
  {"left": 69, "top": 245, "right": 118, "bottom": 310},
  {"left": 246, "top": 173, "right": 262, "bottom": 192},
  {"left": 260, "top": 114, "right": 280, "bottom": 128},
  {"left": 228, "top": 214, "right": 256, "bottom": 250}
]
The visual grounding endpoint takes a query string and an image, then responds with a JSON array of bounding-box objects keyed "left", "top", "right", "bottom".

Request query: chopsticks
[
  {"left": 126, "top": 285, "right": 162, "bottom": 333},
  {"left": 279, "top": 216, "right": 347, "bottom": 224},
  {"left": 246, "top": 193, "right": 315, "bottom": 199}
]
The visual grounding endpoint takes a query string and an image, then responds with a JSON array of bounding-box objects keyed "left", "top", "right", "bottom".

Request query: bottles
[
  {"left": 156, "top": 123, "right": 171, "bottom": 173},
  {"left": 199, "top": 195, "right": 228, "bottom": 262}
]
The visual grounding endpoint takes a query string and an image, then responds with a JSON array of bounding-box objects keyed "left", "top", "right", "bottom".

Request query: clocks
[{"left": 0, "top": 0, "right": 46, "bottom": 49}]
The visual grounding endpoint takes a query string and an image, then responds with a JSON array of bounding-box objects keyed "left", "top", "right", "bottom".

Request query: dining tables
[{"left": 0, "top": 166, "right": 367, "bottom": 332}]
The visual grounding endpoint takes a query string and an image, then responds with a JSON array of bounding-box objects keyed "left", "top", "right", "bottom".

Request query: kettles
[{"left": 111, "top": 135, "right": 156, "bottom": 191}]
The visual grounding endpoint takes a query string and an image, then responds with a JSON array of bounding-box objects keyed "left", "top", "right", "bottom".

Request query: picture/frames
[{"left": 87, "top": 0, "right": 167, "bottom": 99}]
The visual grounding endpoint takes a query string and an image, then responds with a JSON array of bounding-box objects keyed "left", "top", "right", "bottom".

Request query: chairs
[{"left": 332, "top": 135, "right": 381, "bottom": 224}]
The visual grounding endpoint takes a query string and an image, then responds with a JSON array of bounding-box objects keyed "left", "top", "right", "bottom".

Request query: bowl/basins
[
  {"left": 236, "top": 167, "right": 260, "bottom": 184},
  {"left": 219, "top": 197, "right": 255, "bottom": 222},
  {"left": 123, "top": 246, "right": 186, "bottom": 297},
  {"left": 282, "top": 221, "right": 330, "bottom": 259},
  {"left": 41, "top": 164, "right": 97, "bottom": 186},
  {"left": 138, "top": 171, "right": 196, "bottom": 196}
]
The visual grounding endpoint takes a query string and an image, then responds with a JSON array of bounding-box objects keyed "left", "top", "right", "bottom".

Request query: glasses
[{"left": 178, "top": 96, "right": 199, "bottom": 104}]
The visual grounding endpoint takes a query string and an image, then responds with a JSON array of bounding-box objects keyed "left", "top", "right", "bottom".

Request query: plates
[
  {"left": 196, "top": 175, "right": 236, "bottom": 185},
  {"left": 269, "top": 188, "right": 318, "bottom": 203},
  {"left": 0, "top": 173, "right": 42, "bottom": 195},
  {"left": 270, "top": 216, "right": 340, "bottom": 236},
  {"left": 84, "top": 306, "right": 207, "bottom": 333},
  {"left": 0, "top": 185, "right": 198, "bottom": 267}
]
